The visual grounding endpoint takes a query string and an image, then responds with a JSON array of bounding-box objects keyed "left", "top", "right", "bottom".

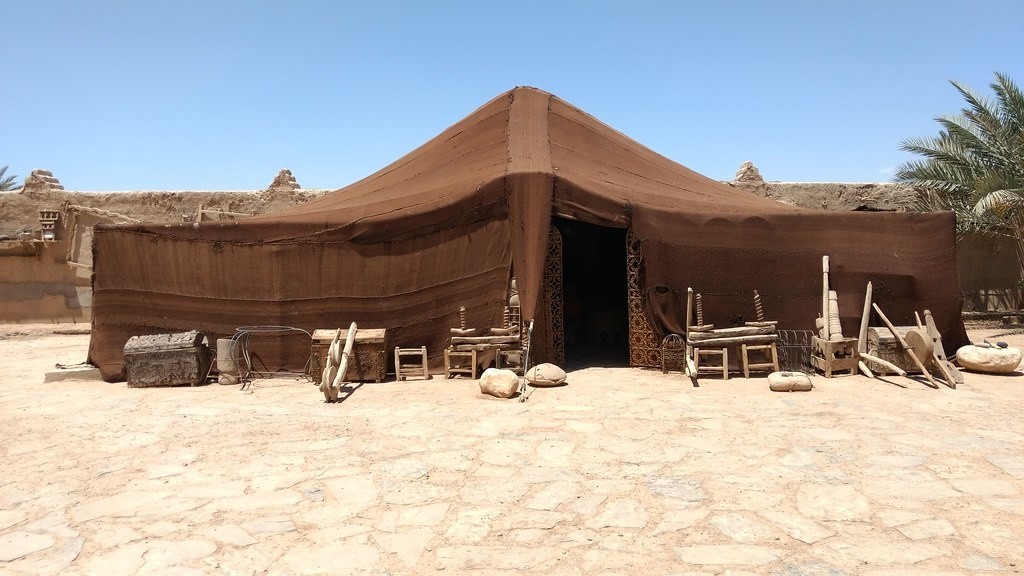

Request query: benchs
[
  {"left": 686, "top": 289, "right": 778, "bottom": 340},
  {"left": 449, "top": 304, "right": 521, "bottom": 344}
]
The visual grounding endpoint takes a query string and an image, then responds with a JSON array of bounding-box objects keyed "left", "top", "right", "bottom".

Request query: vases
[
  {"left": 20, "top": 233, "right": 31, "bottom": 240},
  {"left": 33, "top": 230, "right": 41, "bottom": 239}
]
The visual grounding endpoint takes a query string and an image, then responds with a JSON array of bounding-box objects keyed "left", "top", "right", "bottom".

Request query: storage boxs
[
  {"left": 865, "top": 326, "right": 942, "bottom": 376},
  {"left": 311, "top": 329, "right": 389, "bottom": 383},
  {"left": 123, "top": 330, "right": 211, "bottom": 388}
]
